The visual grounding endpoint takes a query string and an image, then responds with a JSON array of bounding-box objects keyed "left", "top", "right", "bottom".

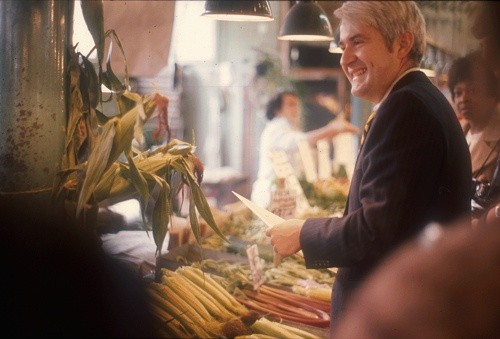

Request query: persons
[
  {"left": 265, "top": 0, "right": 473, "bottom": 339},
  {"left": 250, "top": 90, "right": 360, "bottom": 215},
  {"left": 447, "top": 53, "right": 500, "bottom": 172}
]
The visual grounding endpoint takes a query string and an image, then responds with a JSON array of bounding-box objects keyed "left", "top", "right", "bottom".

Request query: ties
[{"left": 360, "top": 110, "right": 376, "bottom": 148}]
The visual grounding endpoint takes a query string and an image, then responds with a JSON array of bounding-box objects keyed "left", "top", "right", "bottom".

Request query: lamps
[
  {"left": 277, "top": 0, "right": 334, "bottom": 41},
  {"left": 201, "top": 0, "right": 275, "bottom": 22}
]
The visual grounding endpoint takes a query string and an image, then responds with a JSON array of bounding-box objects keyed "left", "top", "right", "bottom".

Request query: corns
[
  {"left": 74, "top": 94, "right": 198, "bottom": 205},
  {"left": 142, "top": 266, "right": 321, "bottom": 339}
]
